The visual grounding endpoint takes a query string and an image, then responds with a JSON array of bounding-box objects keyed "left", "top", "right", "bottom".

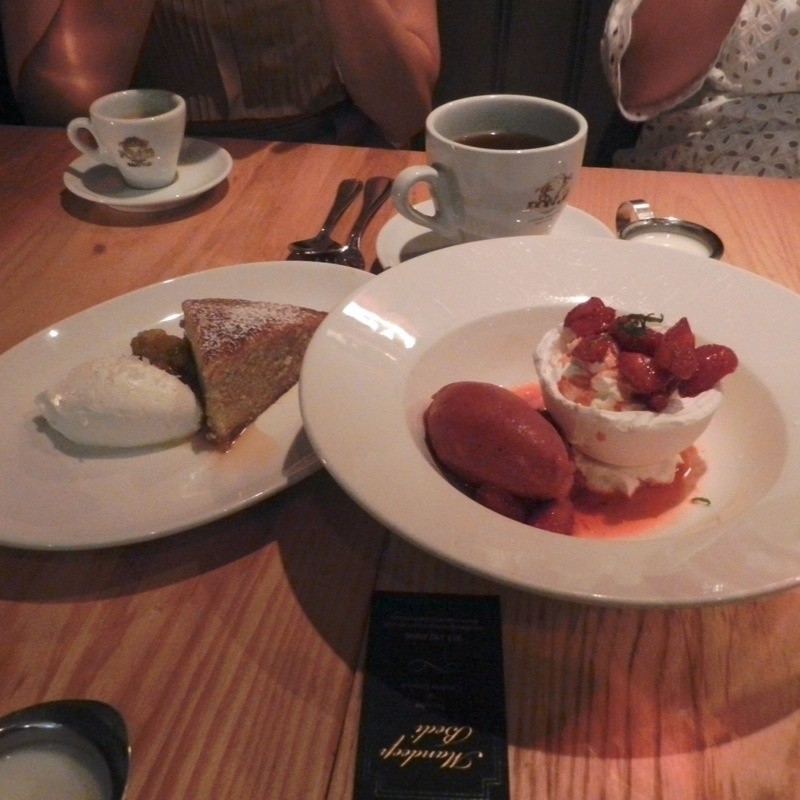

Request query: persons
[
  {"left": 0, "top": 0, "right": 443, "bottom": 150},
  {"left": 598, "top": 0, "right": 800, "bottom": 180}
]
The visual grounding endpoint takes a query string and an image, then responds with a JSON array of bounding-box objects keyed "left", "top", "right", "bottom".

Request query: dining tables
[{"left": 0, "top": 121, "right": 800, "bottom": 800}]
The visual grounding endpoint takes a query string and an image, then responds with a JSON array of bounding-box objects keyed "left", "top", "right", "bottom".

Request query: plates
[
  {"left": 299, "top": 230, "right": 800, "bottom": 603},
  {"left": 376, "top": 197, "right": 615, "bottom": 271},
  {"left": 0, "top": 256, "right": 385, "bottom": 554},
  {"left": 59, "top": 138, "right": 234, "bottom": 211}
]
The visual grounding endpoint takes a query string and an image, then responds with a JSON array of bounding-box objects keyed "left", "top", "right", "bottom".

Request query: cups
[
  {"left": 66, "top": 89, "right": 188, "bottom": 187},
  {"left": 387, "top": 91, "right": 589, "bottom": 244},
  {"left": 0, "top": 697, "right": 129, "bottom": 799},
  {"left": 618, "top": 197, "right": 725, "bottom": 260}
]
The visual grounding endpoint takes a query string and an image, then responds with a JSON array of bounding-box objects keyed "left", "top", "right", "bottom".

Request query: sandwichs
[{"left": 180, "top": 297, "right": 331, "bottom": 453}]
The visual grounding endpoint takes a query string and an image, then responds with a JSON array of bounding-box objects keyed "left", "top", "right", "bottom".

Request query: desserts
[{"left": 532, "top": 296, "right": 739, "bottom": 498}]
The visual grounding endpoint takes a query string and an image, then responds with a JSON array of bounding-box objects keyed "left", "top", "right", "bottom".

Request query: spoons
[{"left": 293, "top": 172, "right": 390, "bottom": 271}]
